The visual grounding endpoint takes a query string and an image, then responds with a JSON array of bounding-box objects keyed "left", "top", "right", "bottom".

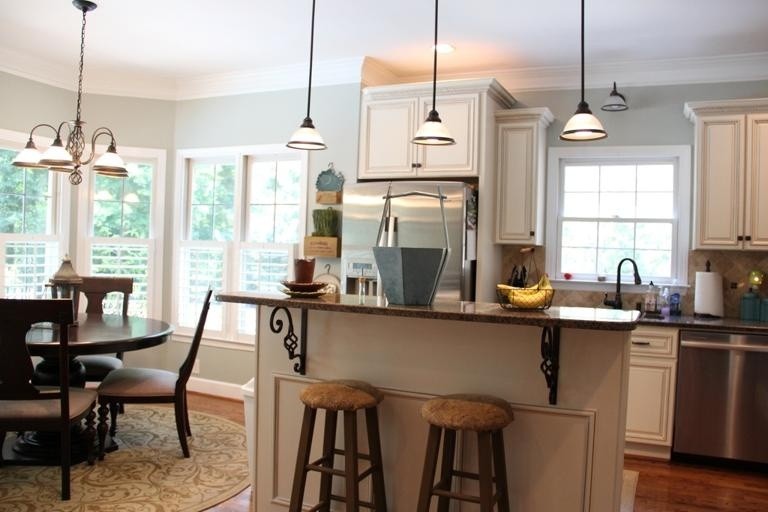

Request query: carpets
[{"left": 0, "top": 405, "right": 251, "bottom": 512}]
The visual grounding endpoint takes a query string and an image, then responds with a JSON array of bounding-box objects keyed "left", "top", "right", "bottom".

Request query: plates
[
  {"left": 280, "top": 289, "right": 326, "bottom": 298},
  {"left": 281, "top": 280, "right": 328, "bottom": 291}
]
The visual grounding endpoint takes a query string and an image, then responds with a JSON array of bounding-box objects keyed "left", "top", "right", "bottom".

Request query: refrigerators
[{"left": 341, "top": 181, "right": 477, "bottom": 301}]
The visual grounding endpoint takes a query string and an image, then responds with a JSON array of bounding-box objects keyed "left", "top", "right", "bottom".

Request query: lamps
[
  {"left": 600, "top": 81, "right": 629, "bottom": 114},
  {"left": 557, "top": 0, "right": 609, "bottom": 143},
  {"left": 285, "top": 0, "right": 329, "bottom": 152},
  {"left": 409, "top": 0, "right": 457, "bottom": 147},
  {"left": 9, "top": 0, "right": 131, "bottom": 187}
]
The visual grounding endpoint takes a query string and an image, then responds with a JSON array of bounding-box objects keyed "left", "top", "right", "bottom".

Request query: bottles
[
  {"left": 670, "top": 279, "right": 682, "bottom": 315},
  {"left": 645, "top": 281, "right": 654, "bottom": 311}
]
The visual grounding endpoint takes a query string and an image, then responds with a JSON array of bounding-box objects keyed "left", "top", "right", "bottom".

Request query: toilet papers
[{"left": 693, "top": 271, "right": 724, "bottom": 317}]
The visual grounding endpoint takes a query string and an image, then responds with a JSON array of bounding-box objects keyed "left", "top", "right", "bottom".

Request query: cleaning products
[{"left": 644, "top": 281, "right": 656, "bottom": 311}]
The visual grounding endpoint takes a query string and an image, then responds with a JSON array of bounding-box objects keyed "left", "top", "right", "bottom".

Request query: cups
[
  {"left": 657, "top": 295, "right": 666, "bottom": 313},
  {"left": 295, "top": 256, "right": 316, "bottom": 283}
]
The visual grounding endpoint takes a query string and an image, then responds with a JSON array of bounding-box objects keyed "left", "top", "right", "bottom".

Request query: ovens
[{"left": 672, "top": 331, "right": 768, "bottom": 464}]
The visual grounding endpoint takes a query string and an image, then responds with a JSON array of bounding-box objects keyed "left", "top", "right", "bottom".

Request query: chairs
[
  {"left": 73, "top": 275, "right": 136, "bottom": 416},
  {"left": 96, "top": 288, "right": 215, "bottom": 462},
  {"left": 1, "top": 296, "right": 98, "bottom": 503}
]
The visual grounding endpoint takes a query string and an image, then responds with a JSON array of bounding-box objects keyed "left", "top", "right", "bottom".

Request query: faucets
[{"left": 603, "top": 258, "right": 640, "bottom": 308}]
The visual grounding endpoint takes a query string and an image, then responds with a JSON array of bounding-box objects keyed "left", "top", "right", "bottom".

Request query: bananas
[{"left": 496, "top": 273, "right": 554, "bottom": 308}]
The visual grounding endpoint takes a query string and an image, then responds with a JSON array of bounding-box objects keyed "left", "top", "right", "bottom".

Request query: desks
[{"left": 0, "top": 313, "right": 176, "bottom": 467}]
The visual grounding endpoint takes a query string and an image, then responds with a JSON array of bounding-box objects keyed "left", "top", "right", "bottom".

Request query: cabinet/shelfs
[
  {"left": 213, "top": 289, "right": 643, "bottom": 512},
  {"left": 492, "top": 106, "right": 557, "bottom": 248},
  {"left": 681, "top": 96, "right": 768, "bottom": 254},
  {"left": 355, "top": 77, "right": 518, "bottom": 185},
  {"left": 624, "top": 325, "right": 681, "bottom": 463}
]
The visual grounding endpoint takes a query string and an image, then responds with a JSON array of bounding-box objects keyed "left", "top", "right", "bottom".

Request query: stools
[
  {"left": 286, "top": 377, "right": 391, "bottom": 512},
  {"left": 412, "top": 392, "right": 517, "bottom": 512}
]
